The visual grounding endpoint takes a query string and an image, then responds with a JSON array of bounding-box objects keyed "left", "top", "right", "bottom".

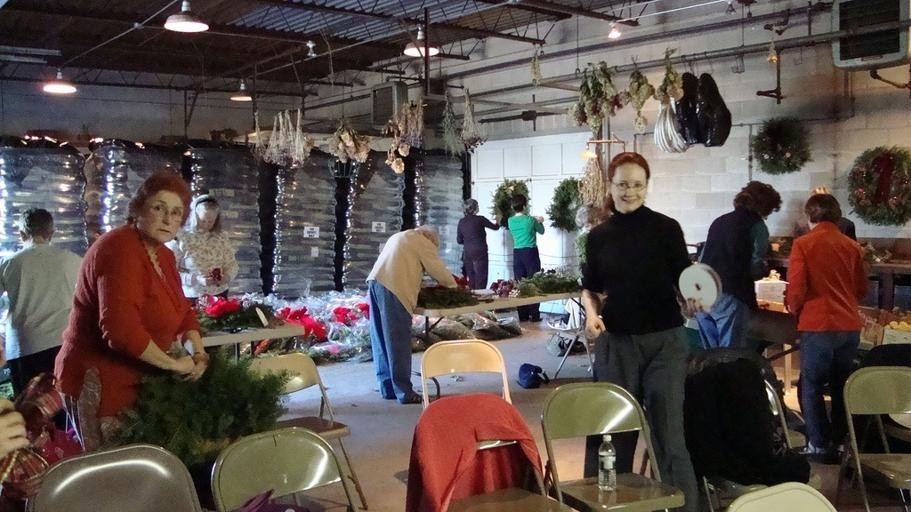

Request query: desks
[
  {"left": 411, "top": 277, "right": 595, "bottom": 402},
  {"left": 186, "top": 316, "right": 306, "bottom": 365}
]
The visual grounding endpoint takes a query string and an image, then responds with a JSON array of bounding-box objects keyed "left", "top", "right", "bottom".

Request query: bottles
[{"left": 599, "top": 434, "right": 616, "bottom": 490}]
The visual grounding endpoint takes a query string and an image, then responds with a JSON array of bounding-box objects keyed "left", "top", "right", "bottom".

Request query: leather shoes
[{"left": 793, "top": 444, "right": 849, "bottom": 466}]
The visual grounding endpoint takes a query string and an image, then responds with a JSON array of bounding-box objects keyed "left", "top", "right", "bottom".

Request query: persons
[
  {"left": 508, "top": 195, "right": 545, "bottom": 322},
  {"left": 169, "top": 194, "right": 239, "bottom": 307},
  {"left": 53, "top": 172, "right": 211, "bottom": 445},
  {"left": 456, "top": 199, "right": 500, "bottom": 290},
  {"left": 366, "top": 225, "right": 458, "bottom": 404},
  {"left": 0, "top": 208, "right": 83, "bottom": 432}
]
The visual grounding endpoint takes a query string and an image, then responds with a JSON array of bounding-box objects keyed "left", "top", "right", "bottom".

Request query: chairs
[
  {"left": 21, "top": 444, "right": 203, "bottom": 512},
  {"left": 210, "top": 353, "right": 370, "bottom": 511},
  {"left": 407, "top": 342, "right": 687, "bottom": 512},
  {"left": 691, "top": 382, "right": 838, "bottom": 511},
  {"left": 841, "top": 344, "right": 911, "bottom": 512}
]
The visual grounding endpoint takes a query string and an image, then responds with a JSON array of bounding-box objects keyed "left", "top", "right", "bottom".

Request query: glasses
[
  {"left": 145, "top": 200, "right": 188, "bottom": 223},
  {"left": 609, "top": 179, "right": 649, "bottom": 192}
]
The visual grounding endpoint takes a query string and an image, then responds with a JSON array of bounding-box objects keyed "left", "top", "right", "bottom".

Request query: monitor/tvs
[
  {"left": 370, "top": 81, "right": 409, "bottom": 130},
  {"left": 830, "top": 0, "right": 911, "bottom": 72}
]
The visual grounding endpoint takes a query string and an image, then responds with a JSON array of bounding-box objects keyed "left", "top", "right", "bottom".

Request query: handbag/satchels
[{"left": 515, "top": 362, "right": 551, "bottom": 389}]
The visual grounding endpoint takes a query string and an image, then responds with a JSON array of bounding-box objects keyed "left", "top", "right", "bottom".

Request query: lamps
[
  {"left": 581, "top": 140, "right": 625, "bottom": 160},
  {"left": 163, "top": 2, "right": 210, "bottom": 34},
  {"left": 39, "top": 65, "right": 77, "bottom": 96},
  {"left": 305, "top": 40, "right": 318, "bottom": 58},
  {"left": 726, "top": 4, "right": 737, "bottom": 16},
  {"left": 401, "top": 23, "right": 440, "bottom": 58},
  {"left": 605, "top": 20, "right": 621, "bottom": 39},
  {"left": 228, "top": 78, "right": 253, "bottom": 101}
]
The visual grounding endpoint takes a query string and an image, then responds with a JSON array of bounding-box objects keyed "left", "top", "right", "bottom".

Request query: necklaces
[{"left": 138, "top": 234, "right": 183, "bottom": 315}]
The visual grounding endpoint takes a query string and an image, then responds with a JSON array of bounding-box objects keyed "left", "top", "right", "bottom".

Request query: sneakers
[
  {"left": 381, "top": 391, "right": 398, "bottom": 400},
  {"left": 399, "top": 392, "right": 432, "bottom": 404}
]
